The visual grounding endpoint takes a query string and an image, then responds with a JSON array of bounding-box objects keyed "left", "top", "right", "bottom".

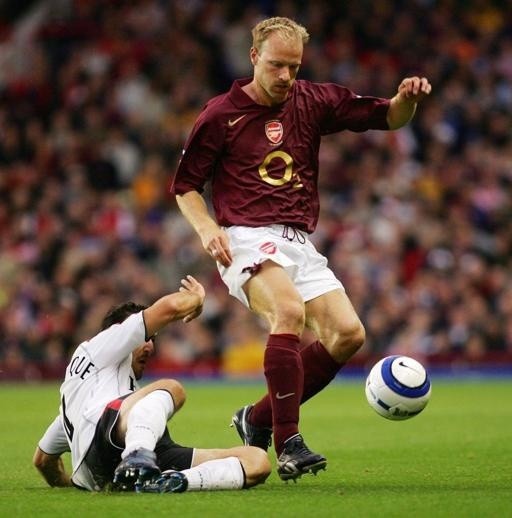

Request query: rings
[{"left": 212, "top": 249, "right": 218, "bottom": 256}]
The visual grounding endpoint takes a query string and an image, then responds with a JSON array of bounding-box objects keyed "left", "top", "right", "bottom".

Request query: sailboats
[{"left": 365, "top": 355, "right": 432, "bottom": 421}]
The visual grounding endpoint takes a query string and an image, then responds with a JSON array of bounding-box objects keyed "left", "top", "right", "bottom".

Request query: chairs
[
  {"left": 232, "top": 404, "right": 272, "bottom": 453},
  {"left": 275, "top": 431, "right": 328, "bottom": 481},
  {"left": 111, "top": 447, "right": 161, "bottom": 492},
  {"left": 134, "top": 468, "right": 188, "bottom": 496}
]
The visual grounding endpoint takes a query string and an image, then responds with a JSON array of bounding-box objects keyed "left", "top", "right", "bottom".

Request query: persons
[
  {"left": 169, "top": 17, "right": 432, "bottom": 484},
  {"left": 34, "top": 273, "right": 271, "bottom": 492},
  {"left": 1, "top": 0, "right": 511, "bottom": 379}
]
